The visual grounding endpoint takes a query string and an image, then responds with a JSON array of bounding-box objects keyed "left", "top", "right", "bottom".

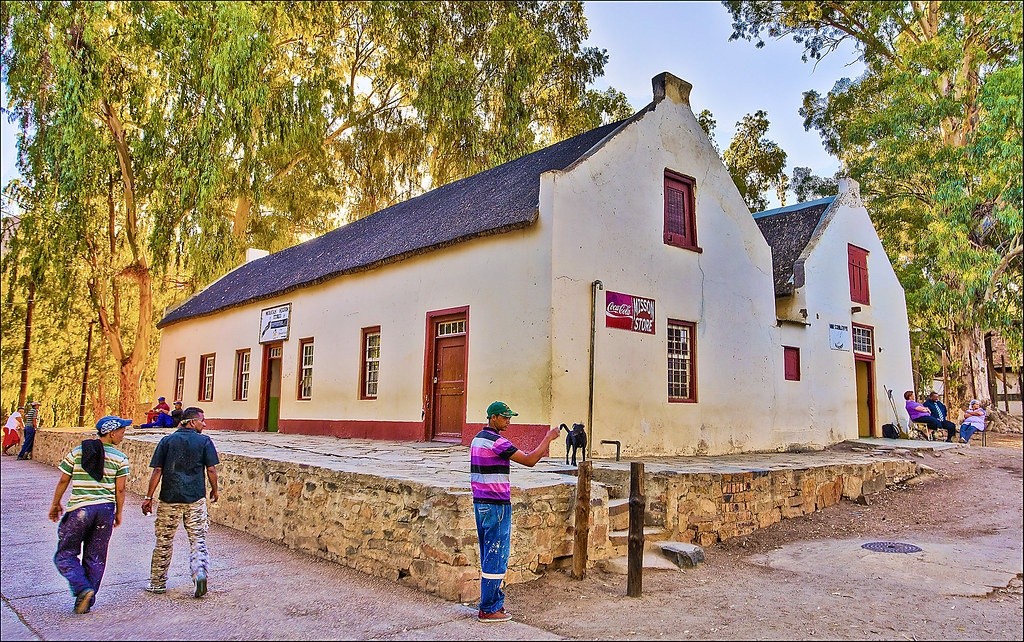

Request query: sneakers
[{"left": 478, "top": 607, "right": 513, "bottom": 622}]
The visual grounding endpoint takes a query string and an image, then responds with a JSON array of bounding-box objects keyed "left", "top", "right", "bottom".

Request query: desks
[{"left": 145, "top": 411, "right": 159, "bottom": 424}]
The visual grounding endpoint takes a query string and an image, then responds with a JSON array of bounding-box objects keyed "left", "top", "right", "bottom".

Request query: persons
[
  {"left": 140, "top": 407, "right": 220, "bottom": 598},
  {"left": 958, "top": 400, "right": 985, "bottom": 443},
  {"left": 16, "top": 402, "right": 42, "bottom": 460},
  {"left": 471, "top": 400, "right": 561, "bottom": 622},
  {"left": 2, "top": 407, "right": 25, "bottom": 456},
  {"left": 133, "top": 396, "right": 184, "bottom": 429},
  {"left": 904, "top": 390, "right": 955, "bottom": 443},
  {"left": 48, "top": 416, "right": 132, "bottom": 614}
]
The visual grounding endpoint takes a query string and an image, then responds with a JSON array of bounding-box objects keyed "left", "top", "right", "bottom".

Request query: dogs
[{"left": 559, "top": 423, "right": 588, "bottom": 466}]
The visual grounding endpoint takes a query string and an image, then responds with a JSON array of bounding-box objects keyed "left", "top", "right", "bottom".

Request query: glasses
[{"left": 181, "top": 418, "right": 205, "bottom": 424}]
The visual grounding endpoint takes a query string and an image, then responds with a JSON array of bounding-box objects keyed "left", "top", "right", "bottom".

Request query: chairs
[
  {"left": 904, "top": 407, "right": 931, "bottom": 442},
  {"left": 959, "top": 416, "right": 992, "bottom": 447}
]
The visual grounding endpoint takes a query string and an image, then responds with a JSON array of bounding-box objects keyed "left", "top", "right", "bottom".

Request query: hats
[
  {"left": 96, "top": 416, "right": 132, "bottom": 434},
  {"left": 487, "top": 401, "right": 518, "bottom": 418},
  {"left": 158, "top": 397, "right": 165, "bottom": 400},
  {"left": 173, "top": 401, "right": 182, "bottom": 406}
]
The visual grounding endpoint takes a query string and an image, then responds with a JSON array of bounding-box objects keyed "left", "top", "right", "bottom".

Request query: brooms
[{"left": 883, "top": 384, "right": 917, "bottom": 440}]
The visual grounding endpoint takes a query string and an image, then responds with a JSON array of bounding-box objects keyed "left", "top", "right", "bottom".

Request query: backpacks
[{"left": 882, "top": 422, "right": 900, "bottom": 439}]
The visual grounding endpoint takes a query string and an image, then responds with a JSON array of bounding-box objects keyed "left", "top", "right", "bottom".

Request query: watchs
[{"left": 144, "top": 496, "right": 152, "bottom": 500}]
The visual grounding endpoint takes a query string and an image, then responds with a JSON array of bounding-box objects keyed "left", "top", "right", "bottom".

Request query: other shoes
[
  {"left": 23, "top": 453, "right": 27, "bottom": 459},
  {"left": 194, "top": 573, "right": 208, "bottom": 598},
  {"left": 31, "top": 402, "right": 41, "bottom": 405},
  {"left": 74, "top": 588, "right": 94, "bottom": 613},
  {"left": 134, "top": 425, "right": 141, "bottom": 428},
  {"left": 922, "top": 433, "right": 928, "bottom": 441},
  {"left": 959, "top": 439, "right": 964, "bottom": 443},
  {"left": 146, "top": 583, "right": 167, "bottom": 594},
  {"left": 17, "top": 457, "right": 23, "bottom": 460}
]
